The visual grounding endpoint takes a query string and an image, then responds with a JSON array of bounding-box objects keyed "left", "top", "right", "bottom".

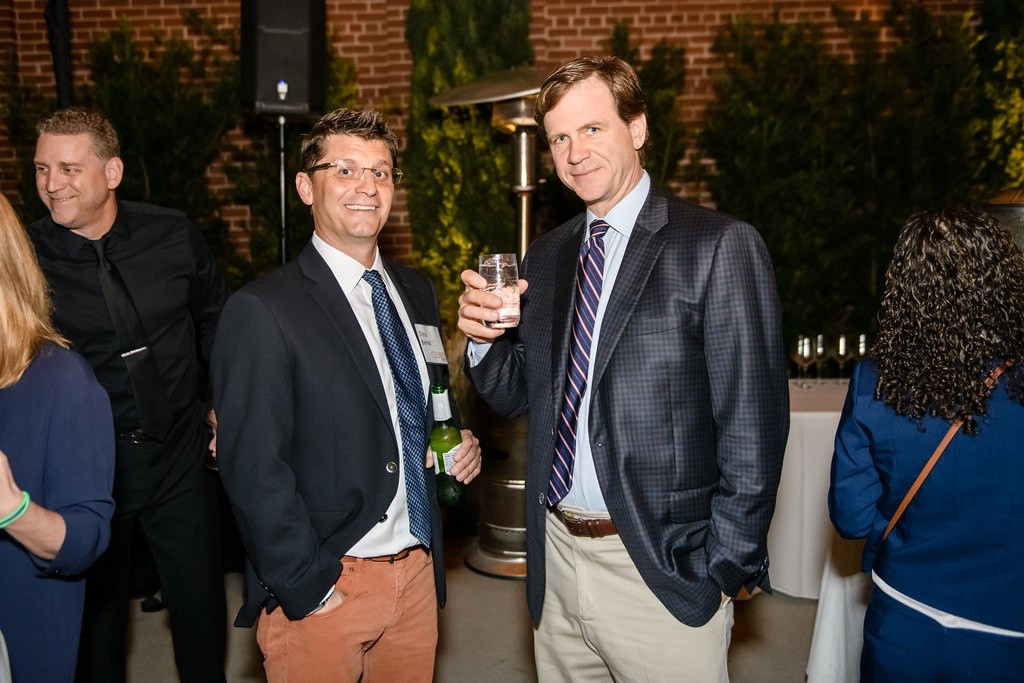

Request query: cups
[{"left": 479, "top": 253, "right": 521, "bottom": 328}]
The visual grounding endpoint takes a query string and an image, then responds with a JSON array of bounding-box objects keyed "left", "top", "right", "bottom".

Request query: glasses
[{"left": 306, "top": 159, "right": 405, "bottom": 186}]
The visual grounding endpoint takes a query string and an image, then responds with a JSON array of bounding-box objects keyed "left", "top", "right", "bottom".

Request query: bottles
[{"left": 430, "top": 383, "right": 470, "bottom": 504}]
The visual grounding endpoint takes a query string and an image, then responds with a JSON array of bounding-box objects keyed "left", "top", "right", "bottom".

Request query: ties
[
  {"left": 546, "top": 219, "right": 612, "bottom": 512},
  {"left": 90, "top": 237, "right": 176, "bottom": 444},
  {"left": 362, "top": 270, "right": 432, "bottom": 549}
]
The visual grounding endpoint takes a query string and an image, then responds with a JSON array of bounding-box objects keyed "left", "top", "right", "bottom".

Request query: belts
[
  {"left": 118, "top": 431, "right": 153, "bottom": 445},
  {"left": 552, "top": 506, "right": 618, "bottom": 539},
  {"left": 339, "top": 544, "right": 422, "bottom": 563}
]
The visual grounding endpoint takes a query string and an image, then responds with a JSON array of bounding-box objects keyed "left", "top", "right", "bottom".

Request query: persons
[
  {"left": 457, "top": 55, "right": 791, "bottom": 683},
  {"left": 26, "top": 106, "right": 228, "bottom": 683},
  {"left": 829, "top": 205, "right": 1024, "bottom": 683},
  {"left": 0, "top": 192, "right": 114, "bottom": 683},
  {"left": 220, "top": 106, "right": 483, "bottom": 683}
]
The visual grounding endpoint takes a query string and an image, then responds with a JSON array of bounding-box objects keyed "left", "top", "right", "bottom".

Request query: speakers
[{"left": 240, "top": 0, "right": 327, "bottom": 123}]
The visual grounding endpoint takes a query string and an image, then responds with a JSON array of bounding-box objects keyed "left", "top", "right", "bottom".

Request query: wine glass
[{"left": 787, "top": 332, "right": 869, "bottom": 380}]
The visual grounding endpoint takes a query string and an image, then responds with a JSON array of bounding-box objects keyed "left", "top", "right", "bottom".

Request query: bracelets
[
  {"left": 0, "top": 491, "right": 30, "bottom": 529},
  {"left": 306, "top": 590, "right": 334, "bottom": 617}
]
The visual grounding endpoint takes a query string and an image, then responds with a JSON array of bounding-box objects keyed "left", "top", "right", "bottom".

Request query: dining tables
[{"left": 767, "top": 378, "right": 850, "bottom": 599}]
